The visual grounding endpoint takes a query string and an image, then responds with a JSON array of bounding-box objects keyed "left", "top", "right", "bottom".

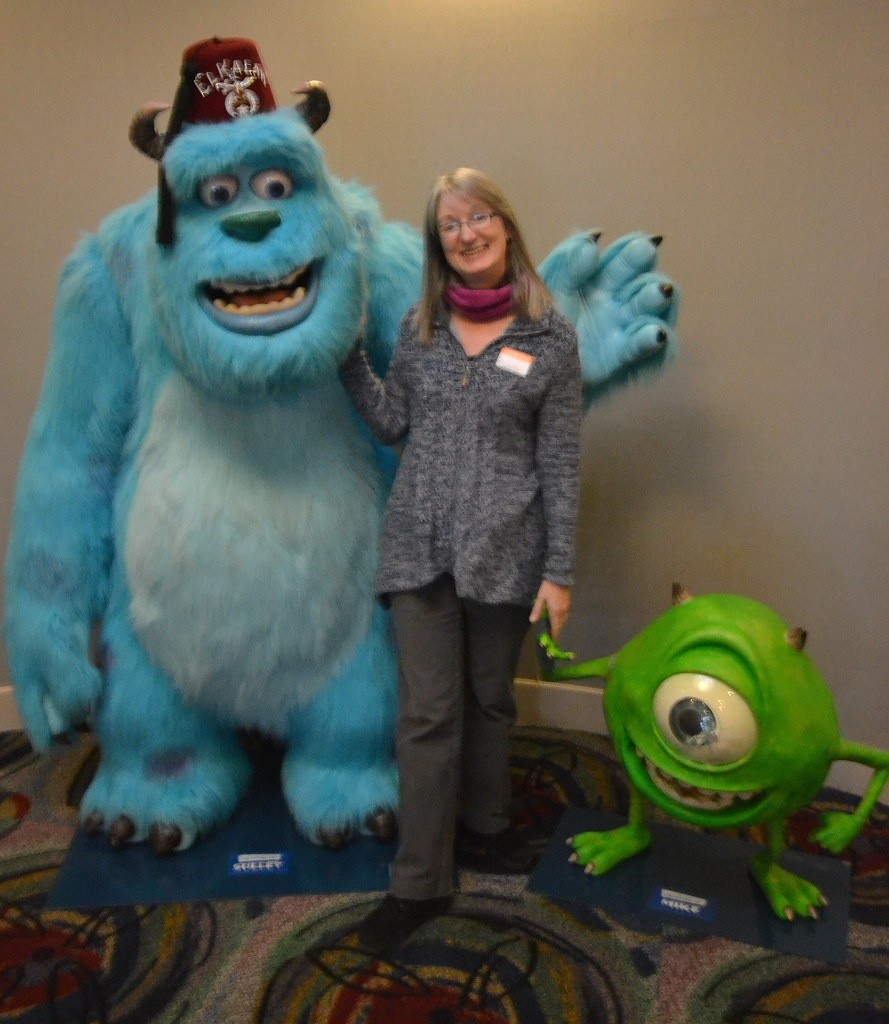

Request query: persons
[{"left": 338, "top": 165, "right": 588, "bottom": 961}]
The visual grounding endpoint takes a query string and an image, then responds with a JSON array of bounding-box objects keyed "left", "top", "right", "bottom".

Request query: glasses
[{"left": 433, "top": 213, "right": 507, "bottom": 237}]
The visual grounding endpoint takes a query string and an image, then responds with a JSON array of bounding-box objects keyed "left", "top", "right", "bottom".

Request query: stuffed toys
[{"left": 0, "top": 33, "right": 678, "bottom": 859}]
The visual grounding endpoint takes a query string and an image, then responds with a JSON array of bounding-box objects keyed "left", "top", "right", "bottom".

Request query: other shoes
[
  {"left": 366, "top": 895, "right": 451, "bottom": 946},
  {"left": 450, "top": 821, "right": 535, "bottom": 866}
]
